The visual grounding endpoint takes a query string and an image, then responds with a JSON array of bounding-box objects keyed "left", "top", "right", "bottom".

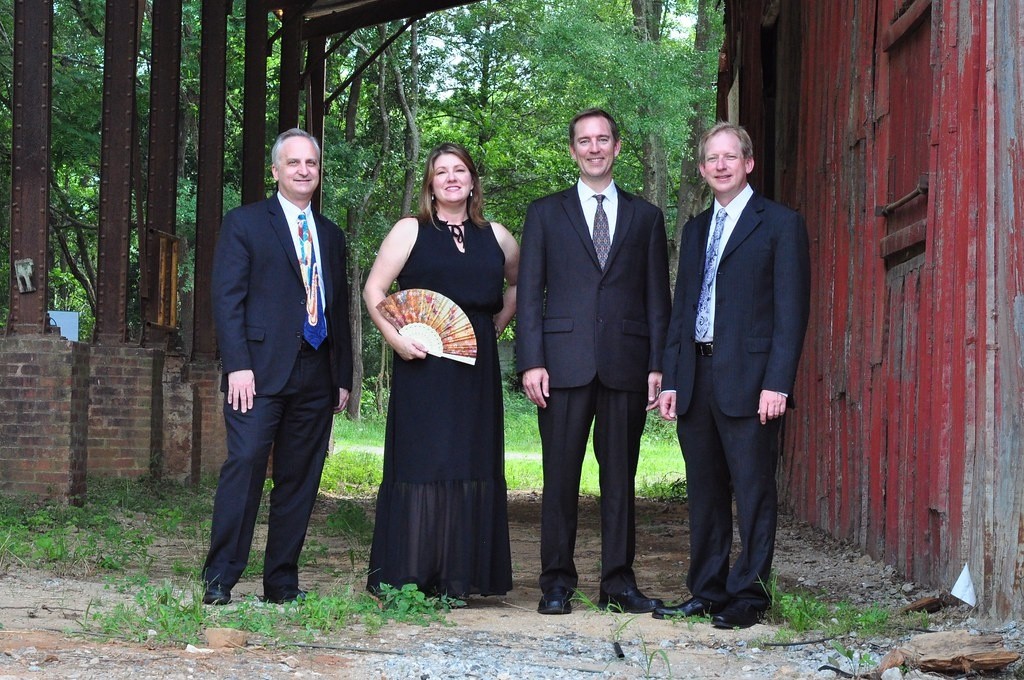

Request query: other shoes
[
  {"left": 204, "top": 582, "right": 230, "bottom": 603},
  {"left": 449, "top": 592, "right": 469, "bottom": 607},
  {"left": 264, "top": 583, "right": 306, "bottom": 604}
]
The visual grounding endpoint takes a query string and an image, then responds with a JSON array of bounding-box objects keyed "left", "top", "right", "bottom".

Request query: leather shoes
[
  {"left": 711, "top": 598, "right": 766, "bottom": 628},
  {"left": 652, "top": 597, "right": 728, "bottom": 619},
  {"left": 598, "top": 583, "right": 664, "bottom": 612},
  {"left": 538, "top": 586, "right": 571, "bottom": 613}
]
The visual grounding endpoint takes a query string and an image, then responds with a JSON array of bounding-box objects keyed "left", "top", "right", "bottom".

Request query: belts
[{"left": 696, "top": 343, "right": 714, "bottom": 357}]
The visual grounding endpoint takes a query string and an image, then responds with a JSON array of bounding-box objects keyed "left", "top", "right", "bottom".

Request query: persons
[
  {"left": 513, "top": 106, "right": 673, "bottom": 616},
  {"left": 363, "top": 141, "right": 521, "bottom": 604},
  {"left": 649, "top": 120, "right": 814, "bottom": 631},
  {"left": 199, "top": 128, "right": 354, "bottom": 603}
]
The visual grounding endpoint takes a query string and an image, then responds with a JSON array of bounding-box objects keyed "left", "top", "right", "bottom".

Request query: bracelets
[{"left": 494, "top": 321, "right": 501, "bottom": 338}]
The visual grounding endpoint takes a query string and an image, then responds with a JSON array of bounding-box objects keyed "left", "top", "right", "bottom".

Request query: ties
[
  {"left": 297, "top": 213, "right": 327, "bottom": 350},
  {"left": 694, "top": 207, "right": 728, "bottom": 343},
  {"left": 592, "top": 194, "right": 611, "bottom": 272}
]
